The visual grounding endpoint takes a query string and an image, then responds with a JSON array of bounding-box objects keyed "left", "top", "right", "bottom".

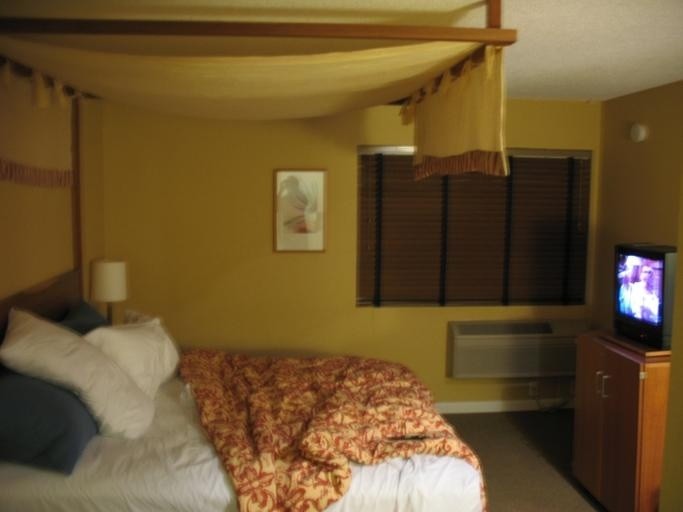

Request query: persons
[{"left": 619, "top": 261, "right": 659, "bottom": 326}]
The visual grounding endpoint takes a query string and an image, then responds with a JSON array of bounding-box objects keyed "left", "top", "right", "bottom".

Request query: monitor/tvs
[{"left": 613, "top": 243, "right": 676, "bottom": 350}]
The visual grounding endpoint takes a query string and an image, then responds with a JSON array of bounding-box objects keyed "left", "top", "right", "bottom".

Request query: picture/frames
[{"left": 272, "top": 167, "right": 327, "bottom": 252}]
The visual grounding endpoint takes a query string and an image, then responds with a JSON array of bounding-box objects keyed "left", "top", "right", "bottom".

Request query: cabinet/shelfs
[{"left": 567, "top": 332, "right": 670, "bottom": 511}]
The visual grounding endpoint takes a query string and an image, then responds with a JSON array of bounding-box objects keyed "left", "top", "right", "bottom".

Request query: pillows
[{"left": 0, "top": 297, "right": 180, "bottom": 477}]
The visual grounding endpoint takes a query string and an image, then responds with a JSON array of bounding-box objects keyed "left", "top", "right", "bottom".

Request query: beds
[{"left": 0, "top": 310, "right": 487, "bottom": 512}]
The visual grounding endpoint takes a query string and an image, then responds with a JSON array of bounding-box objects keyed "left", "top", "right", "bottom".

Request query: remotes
[{"left": 634, "top": 241, "right": 655, "bottom": 248}]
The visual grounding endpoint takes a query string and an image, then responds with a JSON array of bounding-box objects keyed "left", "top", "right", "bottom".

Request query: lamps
[{"left": 89, "top": 257, "right": 129, "bottom": 327}]
